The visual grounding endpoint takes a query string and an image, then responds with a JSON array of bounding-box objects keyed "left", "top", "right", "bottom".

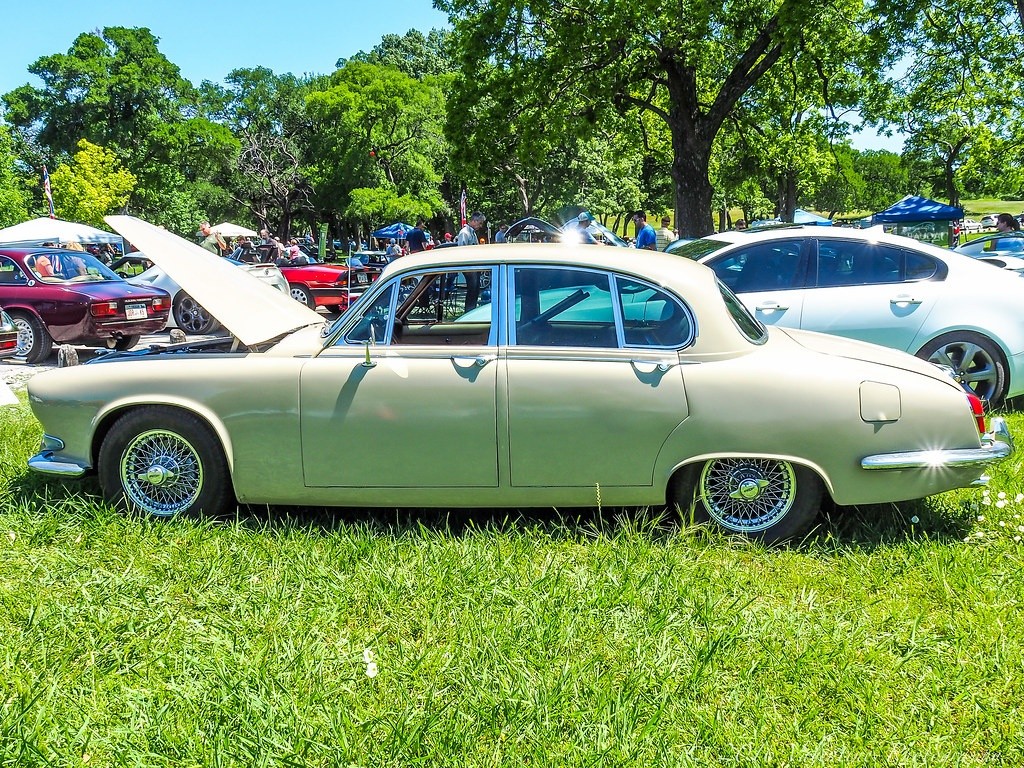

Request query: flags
[
  {"left": 461, "top": 191, "right": 467, "bottom": 229},
  {"left": 44, "top": 165, "right": 55, "bottom": 219}
]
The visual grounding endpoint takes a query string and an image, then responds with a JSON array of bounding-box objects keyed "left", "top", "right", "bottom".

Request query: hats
[
  {"left": 577, "top": 212, "right": 594, "bottom": 222},
  {"left": 662, "top": 218, "right": 670, "bottom": 224},
  {"left": 418, "top": 222, "right": 426, "bottom": 227},
  {"left": 274, "top": 237, "right": 280, "bottom": 240},
  {"left": 445, "top": 233, "right": 452, "bottom": 238},
  {"left": 390, "top": 238, "right": 396, "bottom": 243}
]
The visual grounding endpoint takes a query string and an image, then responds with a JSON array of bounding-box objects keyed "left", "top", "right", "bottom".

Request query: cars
[
  {"left": 26, "top": 214, "right": 1014, "bottom": 548},
  {"left": 978, "top": 214, "right": 1001, "bottom": 228},
  {"left": 225, "top": 241, "right": 490, "bottom": 318},
  {"left": 958, "top": 218, "right": 983, "bottom": 230},
  {"left": 452, "top": 225, "right": 1024, "bottom": 415},
  {"left": 1012, "top": 214, "right": 1024, "bottom": 226},
  {"left": 0, "top": 246, "right": 171, "bottom": 364},
  {"left": 106, "top": 250, "right": 291, "bottom": 336}
]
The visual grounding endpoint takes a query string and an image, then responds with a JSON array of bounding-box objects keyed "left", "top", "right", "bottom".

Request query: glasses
[
  {"left": 501, "top": 227, "right": 506, "bottom": 230},
  {"left": 633, "top": 219, "right": 640, "bottom": 222},
  {"left": 737, "top": 227, "right": 746, "bottom": 230},
  {"left": 997, "top": 221, "right": 999, "bottom": 225}
]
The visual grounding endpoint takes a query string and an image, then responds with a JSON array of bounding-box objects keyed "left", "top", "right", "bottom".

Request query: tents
[
  {"left": 0, "top": 217, "right": 124, "bottom": 270},
  {"left": 369, "top": 222, "right": 430, "bottom": 252},
  {"left": 774, "top": 208, "right": 832, "bottom": 226},
  {"left": 860, "top": 194, "right": 963, "bottom": 250},
  {"left": 196, "top": 223, "right": 258, "bottom": 256},
  {"left": 559, "top": 217, "right": 627, "bottom": 248},
  {"left": 506, "top": 218, "right": 562, "bottom": 244}
]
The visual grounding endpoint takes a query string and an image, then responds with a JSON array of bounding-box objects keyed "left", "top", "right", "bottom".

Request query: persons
[
  {"left": 566, "top": 211, "right": 609, "bottom": 246},
  {"left": 457, "top": 211, "right": 486, "bottom": 246},
  {"left": 222, "top": 228, "right": 314, "bottom": 265},
  {"left": 346, "top": 221, "right": 459, "bottom": 267},
  {"left": 633, "top": 210, "right": 658, "bottom": 251},
  {"left": 653, "top": 216, "right": 676, "bottom": 252},
  {"left": 735, "top": 219, "right": 746, "bottom": 230},
  {"left": 496, "top": 224, "right": 558, "bottom": 244},
  {"left": 199, "top": 221, "right": 226, "bottom": 256},
  {"left": 953, "top": 213, "right": 1024, "bottom": 253},
  {"left": 0, "top": 225, "right": 165, "bottom": 277}
]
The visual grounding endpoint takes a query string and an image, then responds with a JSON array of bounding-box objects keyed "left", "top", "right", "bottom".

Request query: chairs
[
  {"left": 275, "top": 256, "right": 307, "bottom": 266},
  {"left": 0, "top": 270, "right": 21, "bottom": 283},
  {"left": 401, "top": 272, "right": 459, "bottom": 318}
]
